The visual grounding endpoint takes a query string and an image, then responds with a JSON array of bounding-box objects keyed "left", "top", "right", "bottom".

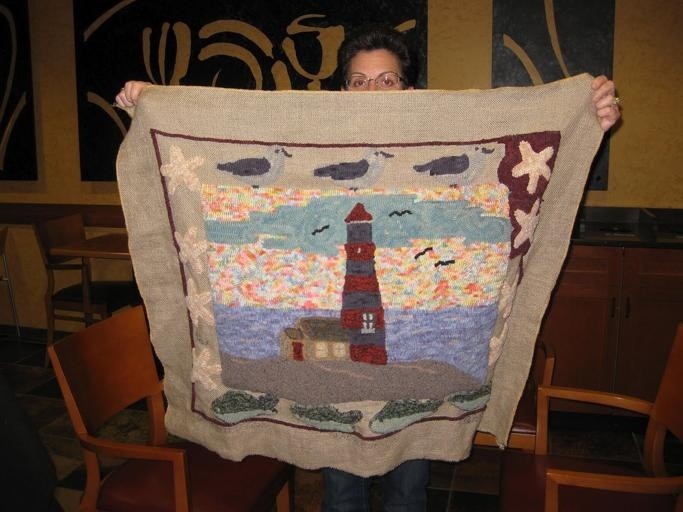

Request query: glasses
[{"left": 346, "top": 72, "right": 409, "bottom": 90}]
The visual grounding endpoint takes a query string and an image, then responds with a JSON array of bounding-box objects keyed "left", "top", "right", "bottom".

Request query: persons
[{"left": 114, "top": 22, "right": 622, "bottom": 512}]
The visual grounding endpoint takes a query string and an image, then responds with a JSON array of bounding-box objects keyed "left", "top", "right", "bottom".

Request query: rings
[
  {"left": 119, "top": 87, "right": 124, "bottom": 92},
  {"left": 611, "top": 95, "right": 620, "bottom": 106}
]
